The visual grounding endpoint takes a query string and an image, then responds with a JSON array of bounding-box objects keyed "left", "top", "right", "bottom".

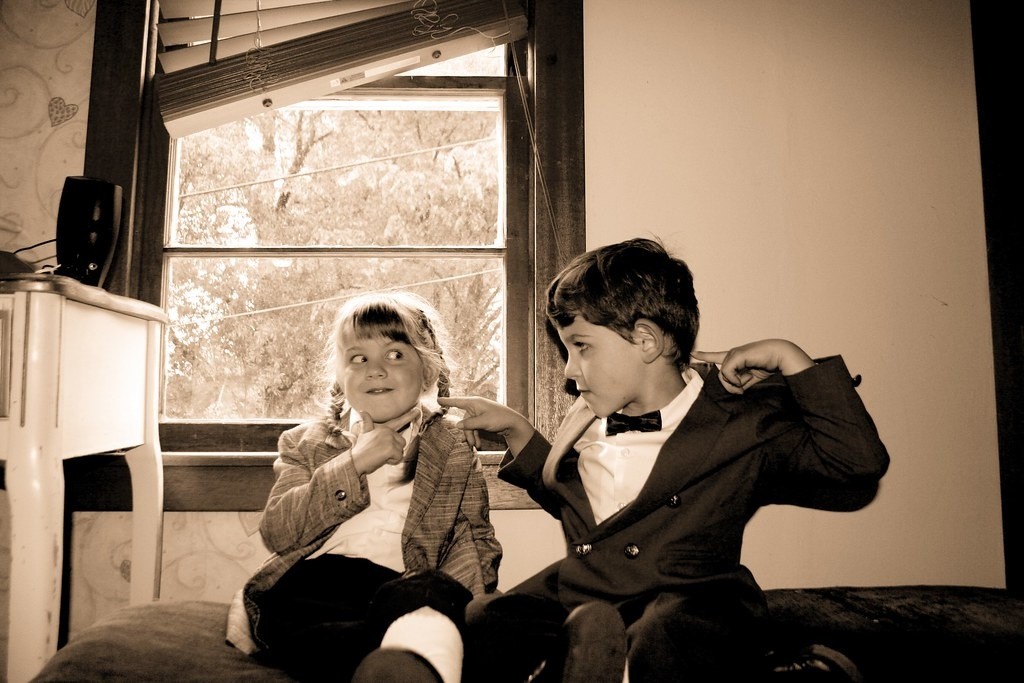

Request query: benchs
[{"left": 36, "top": 584, "right": 1024, "bottom": 683}]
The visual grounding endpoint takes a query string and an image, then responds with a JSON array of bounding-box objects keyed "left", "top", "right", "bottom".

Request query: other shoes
[{"left": 355, "top": 649, "right": 438, "bottom": 683}]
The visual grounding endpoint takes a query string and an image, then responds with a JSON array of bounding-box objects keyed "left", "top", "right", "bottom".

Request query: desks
[{"left": 0, "top": 274, "right": 166, "bottom": 683}]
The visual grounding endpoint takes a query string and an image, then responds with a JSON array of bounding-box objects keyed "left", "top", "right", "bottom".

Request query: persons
[
  {"left": 439, "top": 238, "right": 891, "bottom": 683},
  {"left": 244, "top": 291, "right": 503, "bottom": 683}
]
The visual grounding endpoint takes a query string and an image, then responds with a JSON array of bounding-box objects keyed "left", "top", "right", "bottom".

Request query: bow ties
[{"left": 606, "top": 408, "right": 662, "bottom": 434}]
